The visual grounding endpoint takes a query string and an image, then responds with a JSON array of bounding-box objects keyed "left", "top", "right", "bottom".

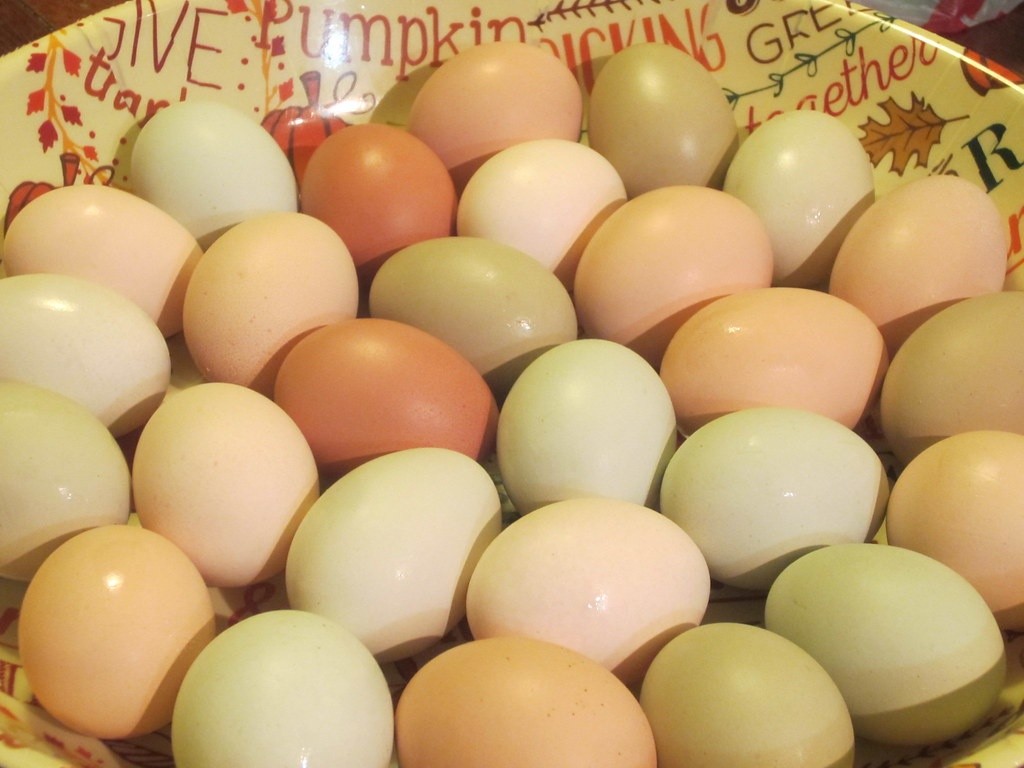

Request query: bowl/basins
[{"left": 0, "top": 0, "right": 1024, "bottom": 768}]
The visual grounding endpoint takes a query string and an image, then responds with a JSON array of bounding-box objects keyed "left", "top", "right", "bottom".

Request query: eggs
[{"left": 0, "top": 40, "right": 1024, "bottom": 765}]
